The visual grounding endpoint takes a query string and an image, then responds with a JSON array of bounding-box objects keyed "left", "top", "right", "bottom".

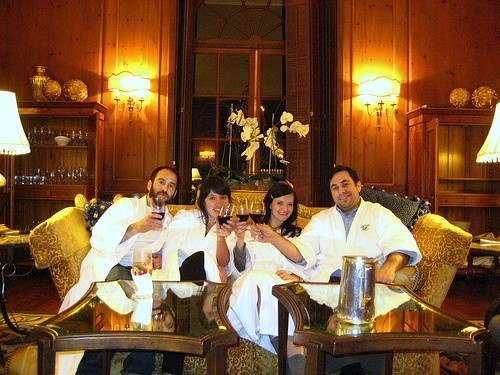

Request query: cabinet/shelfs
[
  {"left": 404, "top": 108, "right": 500, "bottom": 236},
  {"left": 0, "top": 101, "right": 108, "bottom": 230}
]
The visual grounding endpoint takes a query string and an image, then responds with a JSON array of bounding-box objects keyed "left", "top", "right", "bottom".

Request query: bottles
[
  {"left": 25, "top": 125, "right": 88, "bottom": 146},
  {"left": 13, "top": 165, "right": 86, "bottom": 185},
  {"left": 29, "top": 65, "right": 50, "bottom": 101}
]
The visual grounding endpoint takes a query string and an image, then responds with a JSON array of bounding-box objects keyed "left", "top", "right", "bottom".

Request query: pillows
[
  {"left": 84, "top": 198, "right": 112, "bottom": 231},
  {"left": 361, "top": 185, "right": 431, "bottom": 231}
]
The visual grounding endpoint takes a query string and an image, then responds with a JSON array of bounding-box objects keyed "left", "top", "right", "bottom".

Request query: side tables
[{"left": 0, "top": 233, "right": 34, "bottom": 302}]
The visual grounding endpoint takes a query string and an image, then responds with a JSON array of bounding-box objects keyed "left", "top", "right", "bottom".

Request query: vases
[{"left": 30, "top": 64, "right": 50, "bottom": 101}]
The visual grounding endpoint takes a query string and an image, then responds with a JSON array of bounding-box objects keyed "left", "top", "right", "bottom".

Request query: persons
[
  {"left": 75, "top": 166, "right": 180, "bottom": 375},
  {"left": 159, "top": 176, "right": 240, "bottom": 375},
  {"left": 250, "top": 166, "right": 423, "bottom": 375},
  {"left": 231, "top": 179, "right": 321, "bottom": 375}
]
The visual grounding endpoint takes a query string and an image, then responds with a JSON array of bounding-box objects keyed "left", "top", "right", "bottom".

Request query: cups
[
  {"left": 132, "top": 251, "right": 153, "bottom": 274},
  {"left": 149, "top": 196, "right": 165, "bottom": 222}
]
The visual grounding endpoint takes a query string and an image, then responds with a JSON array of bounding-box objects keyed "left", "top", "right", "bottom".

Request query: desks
[{"left": 195, "top": 189, "right": 268, "bottom": 217}]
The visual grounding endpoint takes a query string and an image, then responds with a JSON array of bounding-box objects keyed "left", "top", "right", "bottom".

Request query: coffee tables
[
  {"left": 272, "top": 281, "right": 489, "bottom": 375},
  {"left": 29, "top": 273, "right": 240, "bottom": 375}
]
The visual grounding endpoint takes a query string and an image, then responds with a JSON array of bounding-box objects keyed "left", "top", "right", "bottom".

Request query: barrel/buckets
[{"left": 448, "top": 220, "right": 471, "bottom": 233}]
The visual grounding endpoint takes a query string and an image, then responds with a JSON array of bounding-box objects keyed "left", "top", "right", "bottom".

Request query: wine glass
[{"left": 216, "top": 196, "right": 267, "bottom": 243}]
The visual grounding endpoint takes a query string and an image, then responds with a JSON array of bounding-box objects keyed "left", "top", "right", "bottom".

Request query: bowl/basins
[{"left": 54, "top": 135, "right": 69, "bottom": 146}]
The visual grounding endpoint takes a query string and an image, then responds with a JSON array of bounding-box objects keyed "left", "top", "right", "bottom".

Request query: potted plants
[{"left": 210, "top": 103, "right": 310, "bottom": 190}]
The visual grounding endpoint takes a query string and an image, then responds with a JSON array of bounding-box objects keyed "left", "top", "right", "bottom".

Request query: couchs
[{"left": 27, "top": 196, "right": 471, "bottom": 375}]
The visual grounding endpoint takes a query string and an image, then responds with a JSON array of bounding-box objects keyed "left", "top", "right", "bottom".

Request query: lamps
[
  {"left": 192, "top": 168, "right": 202, "bottom": 181},
  {"left": 0, "top": 90, "right": 31, "bottom": 236},
  {"left": 475, "top": 101, "right": 500, "bottom": 163},
  {"left": 356, "top": 76, "right": 401, "bottom": 132},
  {"left": 108, "top": 70, "right": 151, "bottom": 122}
]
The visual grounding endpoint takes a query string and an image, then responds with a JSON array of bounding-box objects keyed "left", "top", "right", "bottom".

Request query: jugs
[{"left": 335, "top": 255, "right": 379, "bottom": 324}]
